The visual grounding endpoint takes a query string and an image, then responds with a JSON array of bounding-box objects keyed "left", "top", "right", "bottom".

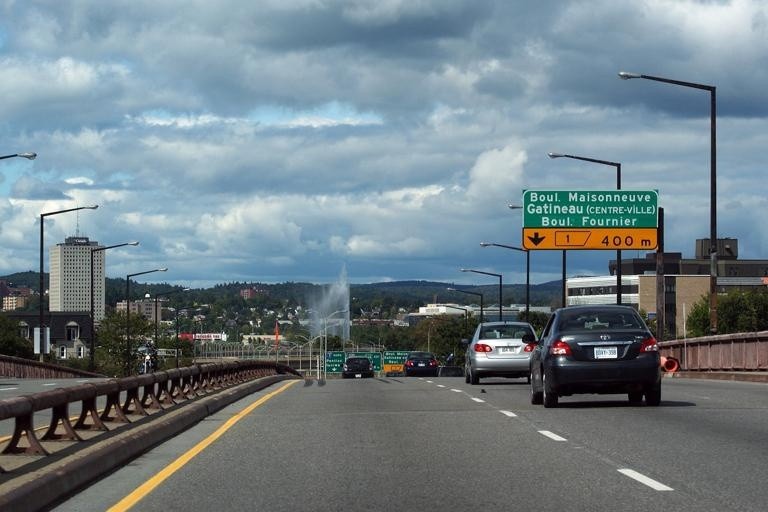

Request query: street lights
[
  {"left": 425, "top": 152, "right": 622, "bottom": 333},
  {"left": 618, "top": 72, "right": 718, "bottom": 339},
  {"left": 202, "top": 310, "right": 348, "bottom": 381}
]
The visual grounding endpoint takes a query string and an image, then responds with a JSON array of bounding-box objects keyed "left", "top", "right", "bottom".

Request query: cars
[
  {"left": 463, "top": 305, "right": 662, "bottom": 405},
  {"left": 403, "top": 351, "right": 437, "bottom": 377},
  {"left": 342, "top": 357, "right": 374, "bottom": 378}
]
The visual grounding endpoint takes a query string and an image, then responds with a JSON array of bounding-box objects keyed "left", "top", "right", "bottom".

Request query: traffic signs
[{"left": 521, "top": 190, "right": 658, "bottom": 247}]
